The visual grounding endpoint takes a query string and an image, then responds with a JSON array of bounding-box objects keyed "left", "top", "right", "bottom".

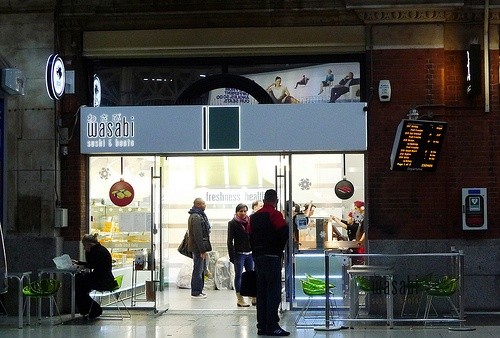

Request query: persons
[
  {"left": 252, "top": 198, "right": 316, "bottom": 249},
  {"left": 69, "top": 233, "right": 114, "bottom": 321},
  {"left": 330, "top": 200, "right": 365, "bottom": 265},
  {"left": 264, "top": 76, "right": 299, "bottom": 105},
  {"left": 328, "top": 69, "right": 361, "bottom": 103},
  {"left": 227, "top": 202, "right": 257, "bottom": 307},
  {"left": 316, "top": 69, "right": 334, "bottom": 95},
  {"left": 251, "top": 189, "right": 290, "bottom": 336},
  {"left": 293, "top": 74, "right": 309, "bottom": 91},
  {"left": 187, "top": 198, "right": 212, "bottom": 299}
]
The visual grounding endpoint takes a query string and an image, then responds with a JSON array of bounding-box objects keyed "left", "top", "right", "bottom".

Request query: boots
[
  {"left": 251, "top": 297, "right": 256, "bottom": 306},
  {"left": 236, "top": 292, "right": 250, "bottom": 307}
]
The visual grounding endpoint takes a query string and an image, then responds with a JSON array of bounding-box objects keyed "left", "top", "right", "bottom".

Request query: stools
[
  {"left": 399, "top": 274, "right": 467, "bottom": 329},
  {"left": 21, "top": 278, "right": 64, "bottom": 326},
  {"left": 294, "top": 273, "right": 340, "bottom": 326},
  {"left": 87, "top": 274, "right": 132, "bottom": 321}
]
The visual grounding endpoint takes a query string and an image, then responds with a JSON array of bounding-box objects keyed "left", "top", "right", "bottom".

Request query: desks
[
  {"left": 346, "top": 263, "right": 395, "bottom": 329},
  {"left": 36, "top": 266, "right": 91, "bottom": 325}
]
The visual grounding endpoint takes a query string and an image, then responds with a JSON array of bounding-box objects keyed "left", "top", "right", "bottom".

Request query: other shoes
[
  {"left": 256, "top": 322, "right": 291, "bottom": 337},
  {"left": 191, "top": 292, "right": 207, "bottom": 299}
]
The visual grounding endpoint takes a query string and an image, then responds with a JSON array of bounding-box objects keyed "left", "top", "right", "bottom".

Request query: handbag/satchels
[
  {"left": 239, "top": 271, "right": 258, "bottom": 297},
  {"left": 177, "top": 232, "right": 192, "bottom": 259}
]
